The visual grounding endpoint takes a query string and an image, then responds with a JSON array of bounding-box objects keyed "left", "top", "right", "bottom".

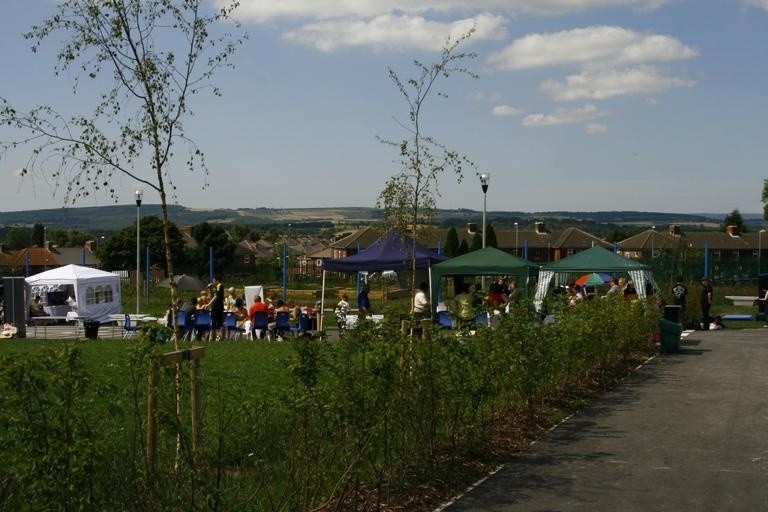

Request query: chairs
[{"left": 122, "top": 305, "right": 499, "bottom": 343}]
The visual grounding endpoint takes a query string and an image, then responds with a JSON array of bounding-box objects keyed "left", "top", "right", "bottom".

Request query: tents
[{"left": 23, "top": 262, "right": 122, "bottom": 327}]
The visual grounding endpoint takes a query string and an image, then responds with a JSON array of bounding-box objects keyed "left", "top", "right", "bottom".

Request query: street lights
[
  {"left": 513, "top": 220, "right": 519, "bottom": 257},
  {"left": 757, "top": 228, "right": 766, "bottom": 276},
  {"left": 477, "top": 171, "right": 491, "bottom": 296},
  {"left": 131, "top": 186, "right": 145, "bottom": 315}
]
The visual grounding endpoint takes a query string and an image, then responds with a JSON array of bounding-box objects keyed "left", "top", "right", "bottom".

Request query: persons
[
  {"left": 165, "top": 281, "right": 311, "bottom": 345},
  {"left": 357, "top": 283, "right": 373, "bottom": 316},
  {"left": 699, "top": 276, "right": 714, "bottom": 331},
  {"left": 335, "top": 293, "right": 351, "bottom": 339},
  {"left": 547, "top": 276, "right": 656, "bottom": 308},
  {"left": 202, "top": 274, "right": 224, "bottom": 342},
  {"left": 31, "top": 295, "right": 50, "bottom": 316},
  {"left": 435, "top": 274, "right": 518, "bottom": 331},
  {"left": 757, "top": 289, "right": 767, "bottom": 328},
  {"left": 410, "top": 281, "right": 431, "bottom": 337},
  {"left": 671, "top": 275, "right": 688, "bottom": 330}
]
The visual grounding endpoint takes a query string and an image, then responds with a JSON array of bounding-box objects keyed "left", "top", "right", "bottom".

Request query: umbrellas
[
  {"left": 157, "top": 273, "right": 208, "bottom": 300},
  {"left": 573, "top": 272, "right": 613, "bottom": 294}
]
